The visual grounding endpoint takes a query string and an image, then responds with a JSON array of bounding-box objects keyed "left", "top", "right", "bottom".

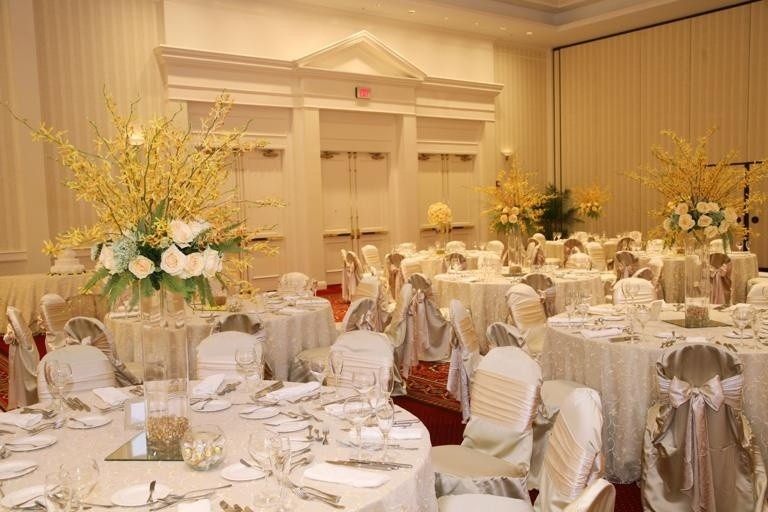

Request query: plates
[
  {"left": 1, "top": 486, "right": 53, "bottom": 508},
  {"left": 110, "top": 483, "right": 170, "bottom": 506}
]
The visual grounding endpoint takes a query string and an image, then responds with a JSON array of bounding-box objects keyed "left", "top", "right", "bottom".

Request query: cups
[{"left": 43, "top": 471, "right": 76, "bottom": 510}]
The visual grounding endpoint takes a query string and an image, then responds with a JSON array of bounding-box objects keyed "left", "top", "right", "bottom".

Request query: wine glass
[
  {"left": 116, "top": 276, "right": 321, "bottom": 325},
  {"left": 248, "top": 428, "right": 290, "bottom": 508},
  {"left": 398, "top": 232, "right": 766, "bottom": 349},
  {"left": 1, "top": 341, "right": 419, "bottom": 480},
  {"left": 61, "top": 458, "right": 99, "bottom": 509}
]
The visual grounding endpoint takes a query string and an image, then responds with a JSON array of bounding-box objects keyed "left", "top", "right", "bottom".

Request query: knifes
[
  {"left": 146, "top": 480, "right": 156, "bottom": 502},
  {"left": 219, "top": 499, "right": 252, "bottom": 511}
]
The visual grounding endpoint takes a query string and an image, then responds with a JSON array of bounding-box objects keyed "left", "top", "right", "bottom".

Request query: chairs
[
  {"left": 432, "top": 346, "right": 544, "bottom": 504},
  {"left": 563, "top": 478, "right": 616, "bottom": 512},
  {"left": 338, "top": 233, "right": 768, "bottom": 364},
  {"left": 445, "top": 298, "right": 485, "bottom": 423},
  {"left": 437, "top": 386, "right": 603, "bottom": 512},
  {"left": 639, "top": 343, "right": 768, "bottom": 511},
  {"left": 6, "top": 231, "right": 768, "bottom": 512},
  {"left": 487, "top": 321, "right": 589, "bottom": 490},
  {"left": 5, "top": 270, "right": 414, "bottom": 401}
]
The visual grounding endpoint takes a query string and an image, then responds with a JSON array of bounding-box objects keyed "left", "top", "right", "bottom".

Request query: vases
[
  {"left": 140, "top": 275, "right": 190, "bottom": 449},
  {"left": 586, "top": 215, "right": 598, "bottom": 234}
]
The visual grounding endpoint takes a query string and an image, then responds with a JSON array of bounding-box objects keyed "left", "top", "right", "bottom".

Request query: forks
[{"left": 158, "top": 484, "right": 236, "bottom": 505}]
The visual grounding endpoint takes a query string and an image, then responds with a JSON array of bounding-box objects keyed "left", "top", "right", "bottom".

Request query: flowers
[
  {"left": 569, "top": 182, "right": 614, "bottom": 219},
  {"left": 471, "top": 154, "right": 556, "bottom": 234},
  {"left": 0, "top": 87, "right": 289, "bottom": 317},
  {"left": 621, "top": 122, "right": 766, "bottom": 251},
  {"left": 428, "top": 201, "right": 453, "bottom": 233}
]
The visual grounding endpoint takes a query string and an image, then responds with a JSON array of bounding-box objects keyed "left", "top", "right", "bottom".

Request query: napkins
[
  {"left": 177, "top": 498, "right": 212, "bottom": 512},
  {"left": 93, "top": 387, "right": 131, "bottom": 406},
  {"left": 0, "top": 410, "right": 43, "bottom": 428},
  {"left": 349, "top": 425, "right": 424, "bottom": 440},
  {"left": 324, "top": 397, "right": 387, "bottom": 419},
  {"left": 303, "top": 461, "right": 404, "bottom": 487}
]
